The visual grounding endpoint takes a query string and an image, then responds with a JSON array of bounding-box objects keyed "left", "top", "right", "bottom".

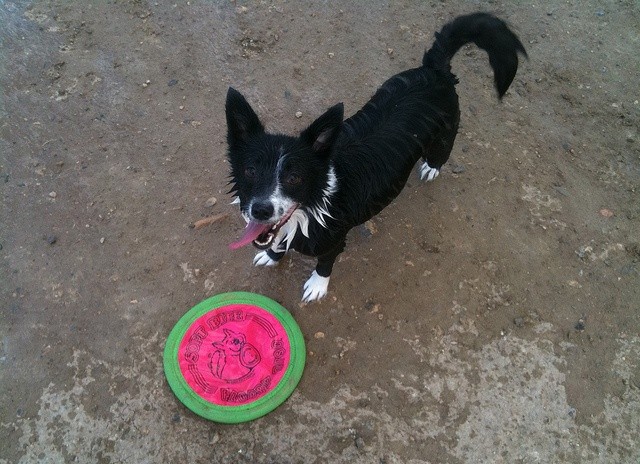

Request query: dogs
[{"left": 223, "top": 10, "right": 529, "bottom": 303}]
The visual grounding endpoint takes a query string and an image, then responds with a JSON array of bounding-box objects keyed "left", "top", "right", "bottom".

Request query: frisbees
[{"left": 164, "top": 292, "right": 307, "bottom": 422}]
